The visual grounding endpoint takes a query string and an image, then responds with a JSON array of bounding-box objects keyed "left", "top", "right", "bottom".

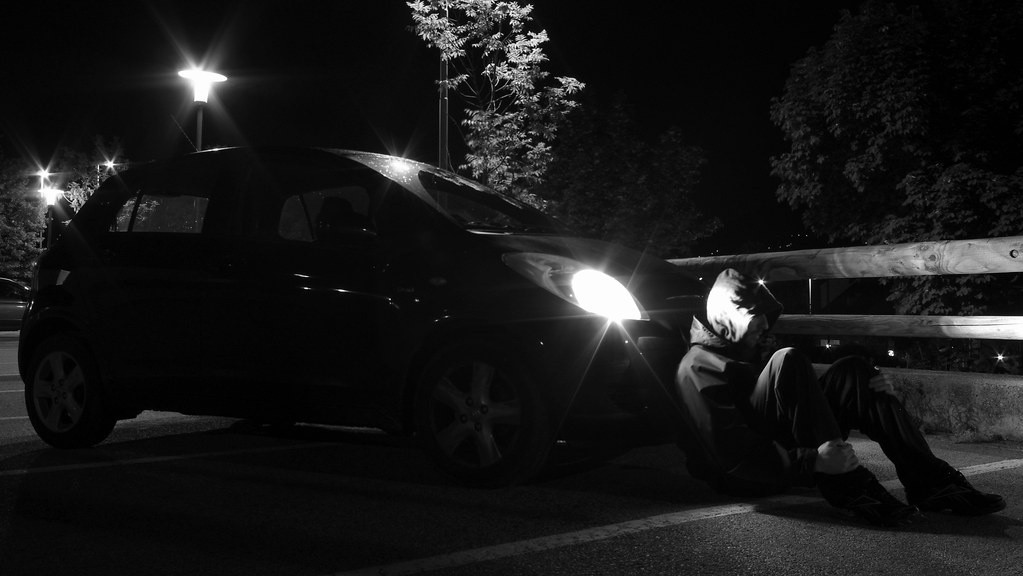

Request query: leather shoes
[
  {"left": 817, "top": 475, "right": 918, "bottom": 527},
  {"left": 905, "top": 466, "right": 1006, "bottom": 515}
]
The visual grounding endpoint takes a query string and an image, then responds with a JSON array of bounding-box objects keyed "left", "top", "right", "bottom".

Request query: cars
[{"left": 16, "top": 141, "right": 710, "bottom": 493}]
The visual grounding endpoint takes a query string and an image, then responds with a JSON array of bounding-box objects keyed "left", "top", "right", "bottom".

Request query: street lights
[
  {"left": 34, "top": 175, "right": 56, "bottom": 249},
  {"left": 174, "top": 61, "right": 228, "bottom": 152}
]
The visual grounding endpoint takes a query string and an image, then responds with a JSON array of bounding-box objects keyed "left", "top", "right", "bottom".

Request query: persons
[{"left": 674, "top": 268, "right": 1006, "bottom": 525}]
